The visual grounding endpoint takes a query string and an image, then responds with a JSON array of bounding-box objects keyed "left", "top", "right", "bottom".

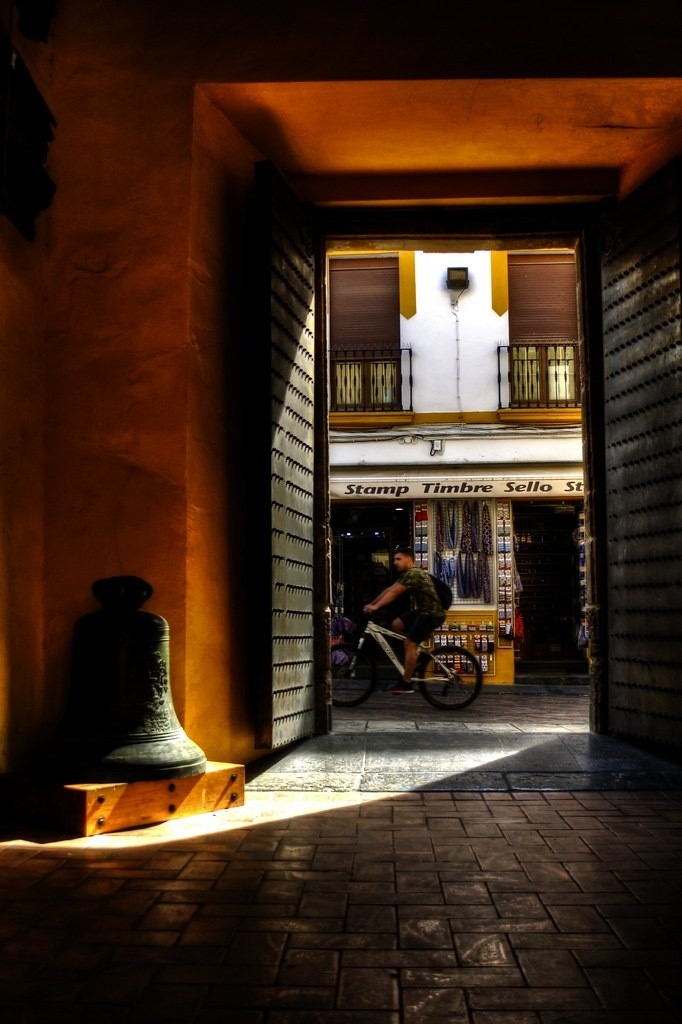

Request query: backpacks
[{"left": 416, "top": 570, "right": 453, "bottom": 610}]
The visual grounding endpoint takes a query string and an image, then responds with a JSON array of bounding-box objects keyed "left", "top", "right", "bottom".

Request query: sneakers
[{"left": 391, "top": 682, "right": 415, "bottom": 694}]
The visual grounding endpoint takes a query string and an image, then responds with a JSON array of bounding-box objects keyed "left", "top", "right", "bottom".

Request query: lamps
[{"left": 446, "top": 267, "right": 469, "bottom": 289}]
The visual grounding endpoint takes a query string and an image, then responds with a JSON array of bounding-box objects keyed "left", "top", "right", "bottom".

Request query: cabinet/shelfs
[{"left": 514, "top": 529, "right": 557, "bottom": 630}]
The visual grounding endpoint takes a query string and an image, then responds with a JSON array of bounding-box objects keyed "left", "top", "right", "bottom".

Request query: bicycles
[{"left": 330, "top": 610, "right": 484, "bottom": 712}]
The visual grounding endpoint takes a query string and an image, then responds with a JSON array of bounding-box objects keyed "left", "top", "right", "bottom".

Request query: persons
[{"left": 363, "top": 547, "right": 452, "bottom": 693}]
